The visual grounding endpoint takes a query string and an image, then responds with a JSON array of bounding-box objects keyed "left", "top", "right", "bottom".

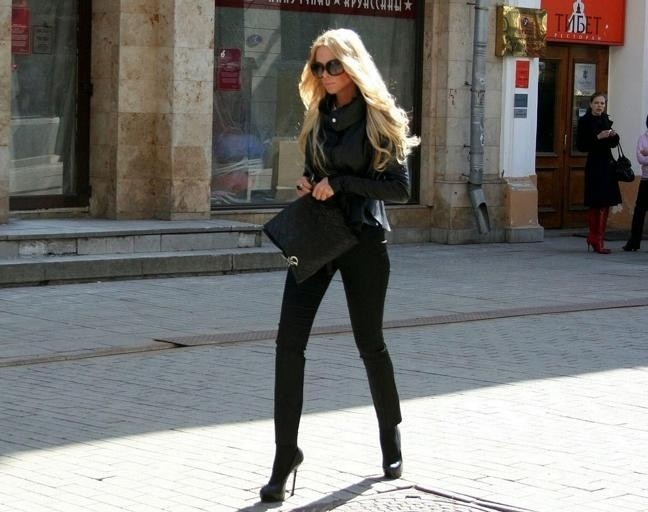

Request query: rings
[{"left": 296, "top": 184, "right": 303, "bottom": 191}]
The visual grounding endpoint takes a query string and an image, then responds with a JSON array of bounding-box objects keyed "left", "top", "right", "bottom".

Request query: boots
[{"left": 587, "top": 208, "right": 611, "bottom": 254}]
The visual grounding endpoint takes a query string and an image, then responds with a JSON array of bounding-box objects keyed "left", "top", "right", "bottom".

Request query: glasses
[{"left": 311, "top": 59, "right": 346, "bottom": 78}]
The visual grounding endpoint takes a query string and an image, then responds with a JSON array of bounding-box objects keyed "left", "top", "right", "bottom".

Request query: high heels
[
  {"left": 260, "top": 446, "right": 304, "bottom": 502},
  {"left": 379, "top": 426, "right": 403, "bottom": 479},
  {"left": 622, "top": 239, "right": 641, "bottom": 251}
]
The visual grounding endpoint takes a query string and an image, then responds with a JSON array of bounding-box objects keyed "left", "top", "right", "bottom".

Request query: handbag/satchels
[
  {"left": 617, "top": 142, "right": 635, "bottom": 182},
  {"left": 262, "top": 191, "right": 360, "bottom": 284}
]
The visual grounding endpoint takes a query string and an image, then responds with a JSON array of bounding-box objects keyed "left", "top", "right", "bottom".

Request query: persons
[
  {"left": 622, "top": 116, "right": 648, "bottom": 252},
  {"left": 259, "top": 27, "right": 411, "bottom": 503},
  {"left": 574, "top": 91, "right": 620, "bottom": 254}
]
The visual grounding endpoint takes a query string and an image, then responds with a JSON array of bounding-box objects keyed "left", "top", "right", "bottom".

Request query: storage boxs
[{"left": 248, "top": 138, "right": 306, "bottom": 201}]
